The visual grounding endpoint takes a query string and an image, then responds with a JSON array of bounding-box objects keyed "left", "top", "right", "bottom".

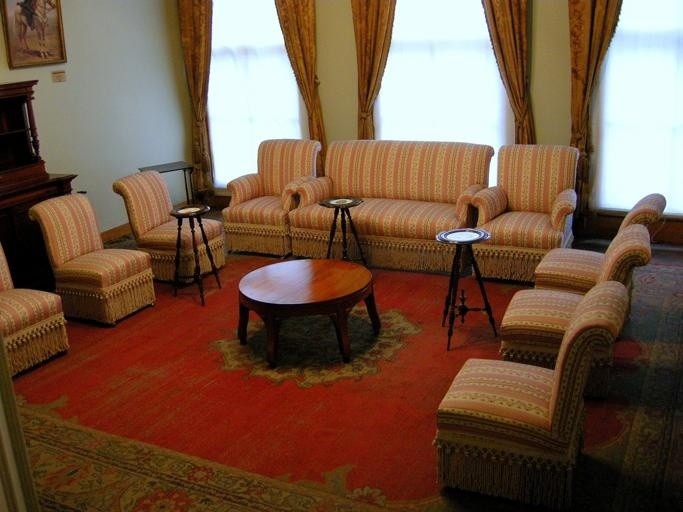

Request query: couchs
[{"left": 288, "top": 137, "right": 496, "bottom": 278}]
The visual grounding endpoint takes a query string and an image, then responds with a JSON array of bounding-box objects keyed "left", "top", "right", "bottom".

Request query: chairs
[
  {"left": 27, "top": 190, "right": 158, "bottom": 327},
  {"left": 112, "top": 169, "right": 226, "bottom": 286},
  {"left": 432, "top": 278, "right": 632, "bottom": 512},
  {"left": 530, "top": 192, "right": 667, "bottom": 292},
  {"left": 496, "top": 224, "right": 651, "bottom": 370},
  {"left": 221, "top": 138, "right": 323, "bottom": 261},
  {"left": 470, "top": 142, "right": 581, "bottom": 286},
  {"left": 1, "top": 245, "right": 70, "bottom": 378}
]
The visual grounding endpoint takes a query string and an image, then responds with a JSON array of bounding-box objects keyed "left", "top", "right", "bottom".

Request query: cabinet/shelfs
[{"left": 0, "top": 78, "right": 79, "bottom": 293}]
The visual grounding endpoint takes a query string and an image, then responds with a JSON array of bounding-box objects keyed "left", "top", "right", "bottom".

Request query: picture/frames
[{"left": 0, "top": 1, "right": 67, "bottom": 70}]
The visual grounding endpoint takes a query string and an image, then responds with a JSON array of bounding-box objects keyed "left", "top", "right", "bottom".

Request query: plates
[
  {"left": 439, "top": 227, "right": 485, "bottom": 244},
  {"left": 321, "top": 196, "right": 360, "bottom": 207},
  {"left": 170, "top": 203, "right": 212, "bottom": 217}
]
